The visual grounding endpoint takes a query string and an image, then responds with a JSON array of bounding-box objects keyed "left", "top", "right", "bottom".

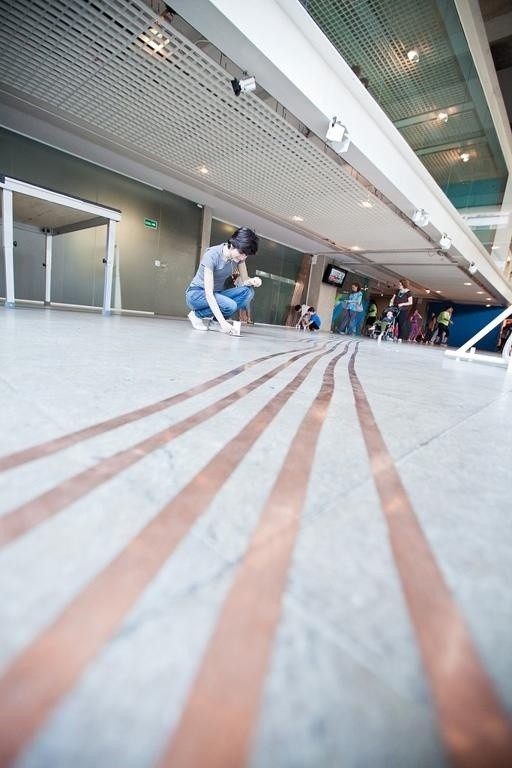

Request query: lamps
[{"left": 230, "top": 71, "right": 257, "bottom": 97}]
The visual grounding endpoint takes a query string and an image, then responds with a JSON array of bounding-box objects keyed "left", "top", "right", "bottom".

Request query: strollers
[{"left": 367, "top": 306, "right": 403, "bottom": 343}]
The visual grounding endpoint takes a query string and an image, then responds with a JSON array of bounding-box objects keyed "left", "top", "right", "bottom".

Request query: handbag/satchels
[{"left": 356, "top": 304, "right": 363, "bottom": 312}]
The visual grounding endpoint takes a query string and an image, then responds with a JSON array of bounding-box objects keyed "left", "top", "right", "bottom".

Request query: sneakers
[
  {"left": 426, "top": 340, "right": 434, "bottom": 345},
  {"left": 441, "top": 342, "right": 448, "bottom": 346},
  {"left": 397, "top": 338, "right": 403, "bottom": 341},
  {"left": 188, "top": 311, "right": 228, "bottom": 334},
  {"left": 408, "top": 339, "right": 417, "bottom": 342}
]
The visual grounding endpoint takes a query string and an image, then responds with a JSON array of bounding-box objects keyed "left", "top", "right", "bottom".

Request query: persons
[
  {"left": 366, "top": 278, "right": 454, "bottom": 347},
  {"left": 294, "top": 303, "right": 315, "bottom": 330},
  {"left": 184, "top": 226, "right": 265, "bottom": 336},
  {"left": 334, "top": 281, "right": 363, "bottom": 337},
  {"left": 497, "top": 319, "right": 511, "bottom": 352},
  {"left": 302, "top": 306, "right": 321, "bottom": 332}
]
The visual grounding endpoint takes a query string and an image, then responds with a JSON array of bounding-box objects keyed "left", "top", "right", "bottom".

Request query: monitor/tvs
[{"left": 322, "top": 263, "right": 348, "bottom": 289}]
